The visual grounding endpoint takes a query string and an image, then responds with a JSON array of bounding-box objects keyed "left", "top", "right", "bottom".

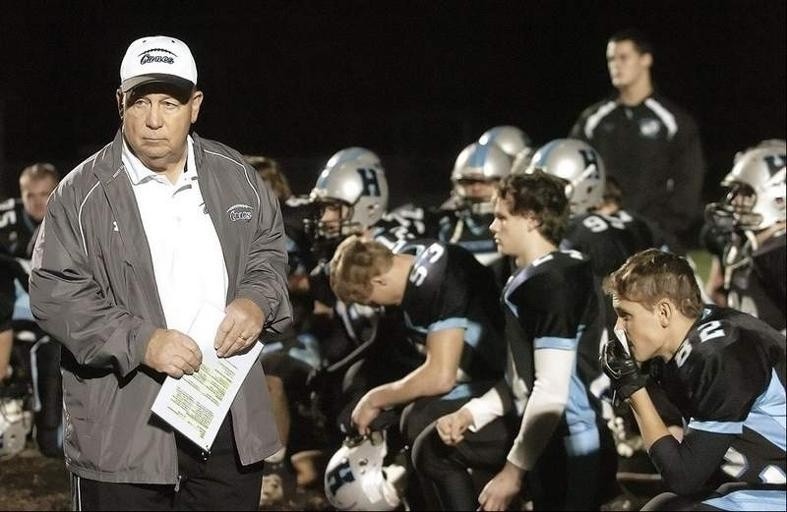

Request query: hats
[{"left": 117, "top": 32, "right": 200, "bottom": 106}]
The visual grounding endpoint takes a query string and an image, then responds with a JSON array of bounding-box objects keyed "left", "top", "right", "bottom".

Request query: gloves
[{"left": 599, "top": 328, "right": 645, "bottom": 400}]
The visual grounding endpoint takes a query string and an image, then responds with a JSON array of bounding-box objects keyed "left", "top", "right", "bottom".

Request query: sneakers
[{"left": 256, "top": 474, "right": 285, "bottom": 509}]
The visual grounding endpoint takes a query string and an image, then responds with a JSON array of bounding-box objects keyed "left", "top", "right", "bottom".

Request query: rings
[{"left": 238, "top": 334, "right": 248, "bottom": 343}]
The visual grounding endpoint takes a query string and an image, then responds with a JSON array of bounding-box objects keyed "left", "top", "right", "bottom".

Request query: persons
[
  {"left": 32, "top": 36, "right": 297, "bottom": 512},
  {"left": 249, "top": 32, "right": 786, "bottom": 512},
  {"left": 1, "top": 165, "right": 64, "bottom": 459},
  {"left": 0, "top": 247, "right": 16, "bottom": 380}
]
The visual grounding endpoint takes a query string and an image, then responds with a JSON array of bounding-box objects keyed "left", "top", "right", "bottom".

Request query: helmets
[
  {"left": 448, "top": 139, "right": 513, "bottom": 217},
  {"left": 313, "top": 161, "right": 388, "bottom": 237},
  {"left": 476, "top": 123, "right": 533, "bottom": 174},
  {"left": 323, "top": 427, "right": 402, "bottom": 512},
  {"left": 721, "top": 140, "right": 787, "bottom": 233},
  {"left": 324, "top": 146, "right": 383, "bottom": 168},
  {"left": 526, "top": 137, "right": 608, "bottom": 225}
]
